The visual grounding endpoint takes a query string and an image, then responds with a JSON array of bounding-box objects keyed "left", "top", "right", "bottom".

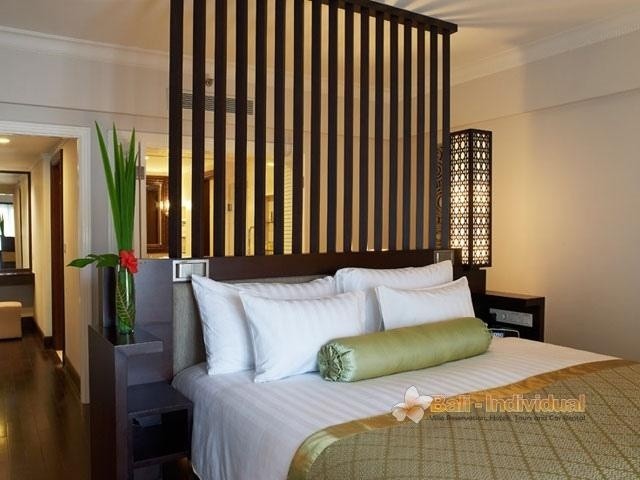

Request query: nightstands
[{"left": 86, "top": 325, "right": 196, "bottom": 479}]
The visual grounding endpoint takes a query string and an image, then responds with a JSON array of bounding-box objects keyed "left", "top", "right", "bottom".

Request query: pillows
[
  {"left": 318, "top": 318, "right": 491, "bottom": 383},
  {"left": 337, "top": 260, "right": 453, "bottom": 333},
  {"left": 376, "top": 276, "right": 476, "bottom": 333},
  {"left": 238, "top": 292, "right": 362, "bottom": 383},
  {"left": 191, "top": 275, "right": 334, "bottom": 376}
]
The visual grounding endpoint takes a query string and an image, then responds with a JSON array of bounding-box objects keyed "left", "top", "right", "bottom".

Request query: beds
[{"left": 173, "top": 276, "right": 640, "bottom": 480}]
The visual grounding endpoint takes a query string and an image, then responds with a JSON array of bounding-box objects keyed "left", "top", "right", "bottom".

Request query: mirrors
[{"left": 145, "top": 174, "right": 171, "bottom": 256}]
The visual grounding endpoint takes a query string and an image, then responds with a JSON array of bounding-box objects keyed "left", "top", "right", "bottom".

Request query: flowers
[{"left": 65, "top": 121, "right": 141, "bottom": 334}]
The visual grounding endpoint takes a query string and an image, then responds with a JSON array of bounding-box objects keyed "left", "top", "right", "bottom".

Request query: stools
[{"left": 0, "top": 301, "right": 22, "bottom": 339}]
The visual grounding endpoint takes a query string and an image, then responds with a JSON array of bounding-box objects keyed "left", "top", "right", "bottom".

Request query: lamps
[{"left": 451, "top": 129, "right": 492, "bottom": 268}]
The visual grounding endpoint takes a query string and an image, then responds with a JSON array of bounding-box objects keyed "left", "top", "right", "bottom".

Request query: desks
[{"left": 1, "top": 171, "right": 32, "bottom": 271}]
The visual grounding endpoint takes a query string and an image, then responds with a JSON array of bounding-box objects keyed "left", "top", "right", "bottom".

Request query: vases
[{"left": 117, "top": 264, "right": 135, "bottom": 336}]
